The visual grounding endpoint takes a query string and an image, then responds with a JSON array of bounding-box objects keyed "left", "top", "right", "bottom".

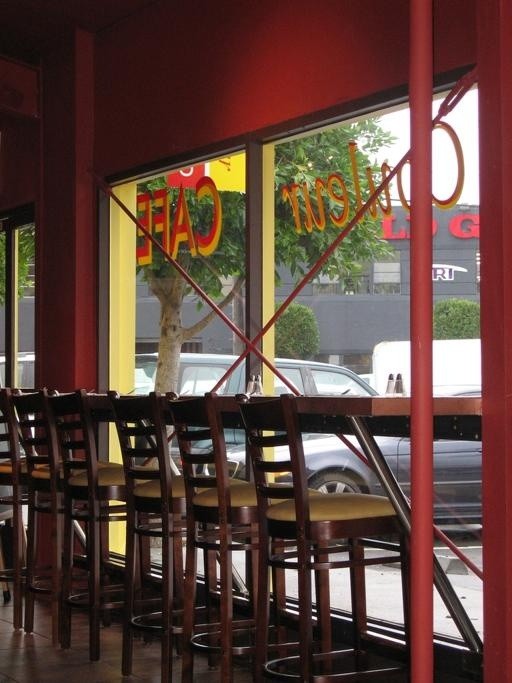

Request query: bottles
[
  {"left": 246, "top": 373, "right": 254, "bottom": 394},
  {"left": 252, "top": 374, "right": 263, "bottom": 396},
  {"left": 393, "top": 373, "right": 404, "bottom": 393},
  {"left": 385, "top": 372, "right": 396, "bottom": 394}
]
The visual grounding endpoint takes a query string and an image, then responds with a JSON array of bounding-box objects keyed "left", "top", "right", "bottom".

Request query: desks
[{"left": 92, "top": 389, "right": 481, "bottom": 680}]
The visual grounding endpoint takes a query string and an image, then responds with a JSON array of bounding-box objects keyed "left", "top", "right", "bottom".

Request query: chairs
[
  {"left": 39, "top": 384, "right": 222, "bottom": 681},
  {"left": 9, "top": 383, "right": 151, "bottom": 652},
  {"left": 161, "top": 386, "right": 337, "bottom": 681},
  {"left": 231, "top": 389, "right": 412, "bottom": 683},
  {"left": 0, "top": 383, "right": 48, "bottom": 630},
  {"left": 103, "top": 384, "right": 290, "bottom": 681}
]
